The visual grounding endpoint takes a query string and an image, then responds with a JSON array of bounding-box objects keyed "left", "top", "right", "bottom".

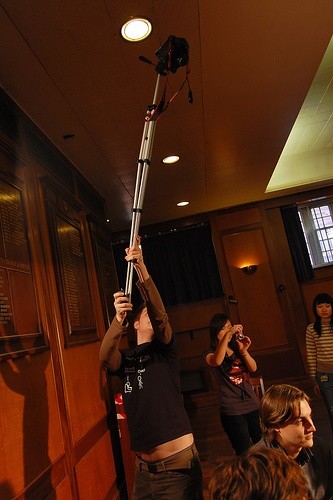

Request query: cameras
[
  {"left": 155, "top": 36, "right": 191, "bottom": 75},
  {"left": 236, "top": 331, "right": 243, "bottom": 343}
]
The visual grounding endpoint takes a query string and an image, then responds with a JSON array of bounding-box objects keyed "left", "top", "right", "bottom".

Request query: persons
[
  {"left": 306, "top": 293, "right": 333, "bottom": 417},
  {"left": 208, "top": 446, "right": 307, "bottom": 500},
  {"left": 203, "top": 313, "right": 262, "bottom": 457},
  {"left": 250, "top": 384, "right": 333, "bottom": 500},
  {"left": 99, "top": 236, "right": 203, "bottom": 500}
]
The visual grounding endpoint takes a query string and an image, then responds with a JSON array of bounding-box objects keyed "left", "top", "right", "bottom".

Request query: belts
[{"left": 135, "top": 446, "right": 197, "bottom": 473}]
